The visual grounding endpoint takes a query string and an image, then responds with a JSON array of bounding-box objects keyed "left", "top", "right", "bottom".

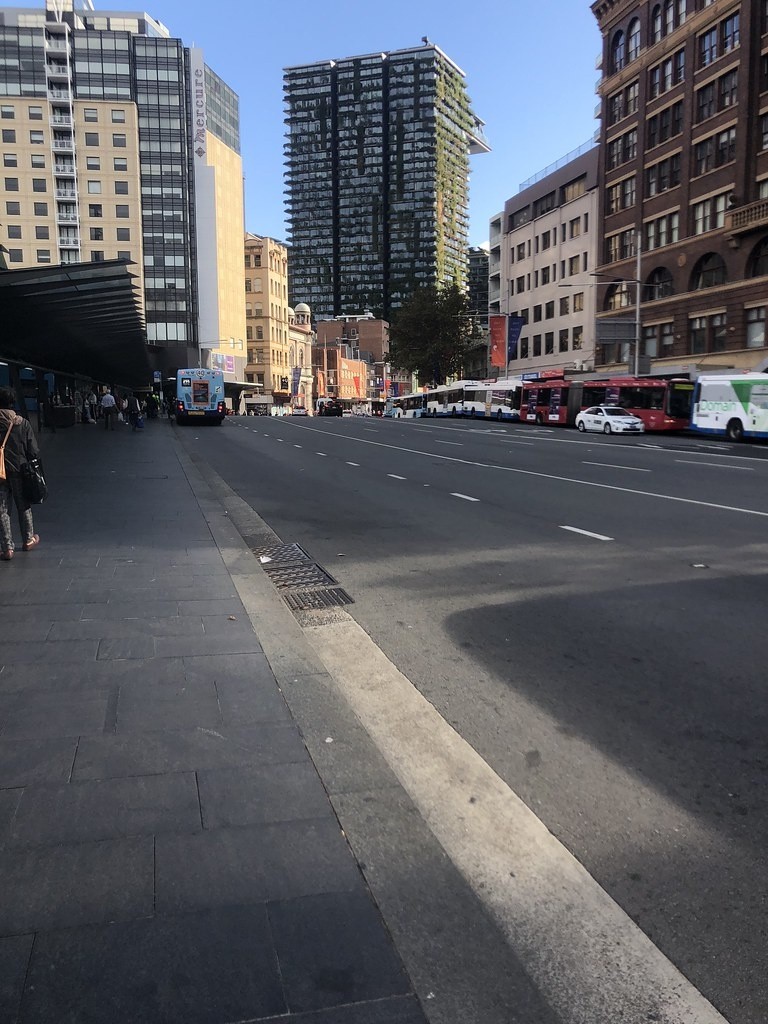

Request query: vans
[{"left": 316, "top": 398, "right": 333, "bottom": 416}]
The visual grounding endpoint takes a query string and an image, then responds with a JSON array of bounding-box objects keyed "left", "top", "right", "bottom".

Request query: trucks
[{"left": 177, "top": 368, "right": 226, "bottom": 425}]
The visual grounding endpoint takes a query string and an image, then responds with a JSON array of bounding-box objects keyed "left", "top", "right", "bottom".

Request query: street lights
[{"left": 558, "top": 272, "right": 662, "bottom": 376}]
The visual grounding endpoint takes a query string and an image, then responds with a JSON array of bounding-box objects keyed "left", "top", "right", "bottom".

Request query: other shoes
[
  {"left": 4, "top": 549, "right": 15, "bottom": 560},
  {"left": 104, "top": 427, "right": 108, "bottom": 430},
  {"left": 111, "top": 428, "right": 114, "bottom": 431},
  {"left": 22, "top": 533, "right": 40, "bottom": 552}
]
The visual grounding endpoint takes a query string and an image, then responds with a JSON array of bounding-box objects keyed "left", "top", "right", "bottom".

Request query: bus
[
  {"left": 520, "top": 376, "right": 695, "bottom": 430},
  {"left": 386, "top": 392, "right": 427, "bottom": 419},
  {"left": 463, "top": 380, "right": 533, "bottom": 421},
  {"left": 426, "top": 380, "right": 481, "bottom": 418},
  {"left": 689, "top": 372, "right": 768, "bottom": 440}
]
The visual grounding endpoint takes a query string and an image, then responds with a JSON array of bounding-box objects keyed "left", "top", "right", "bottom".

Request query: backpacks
[{"left": 20, "top": 456, "right": 49, "bottom": 505}]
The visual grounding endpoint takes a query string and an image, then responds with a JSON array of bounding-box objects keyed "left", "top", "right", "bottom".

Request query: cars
[
  {"left": 322, "top": 401, "right": 343, "bottom": 417},
  {"left": 292, "top": 406, "right": 308, "bottom": 416},
  {"left": 343, "top": 410, "right": 352, "bottom": 417},
  {"left": 575, "top": 403, "right": 645, "bottom": 435}
]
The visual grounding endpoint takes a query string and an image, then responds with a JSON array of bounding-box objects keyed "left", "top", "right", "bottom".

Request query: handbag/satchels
[
  {"left": 0, "top": 446, "right": 6, "bottom": 480},
  {"left": 118, "top": 412, "right": 123, "bottom": 422},
  {"left": 137, "top": 414, "right": 144, "bottom": 428}
]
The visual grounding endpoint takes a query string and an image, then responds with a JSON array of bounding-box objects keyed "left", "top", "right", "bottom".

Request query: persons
[
  {"left": 0, "top": 387, "right": 39, "bottom": 561},
  {"left": 243, "top": 411, "right": 246, "bottom": 416},
  {"left": 83, "top": 387, "right": 157, "bottom": 432},
  {"left": 251, "top": 409, "right": 271, "bottom": 416}
]
